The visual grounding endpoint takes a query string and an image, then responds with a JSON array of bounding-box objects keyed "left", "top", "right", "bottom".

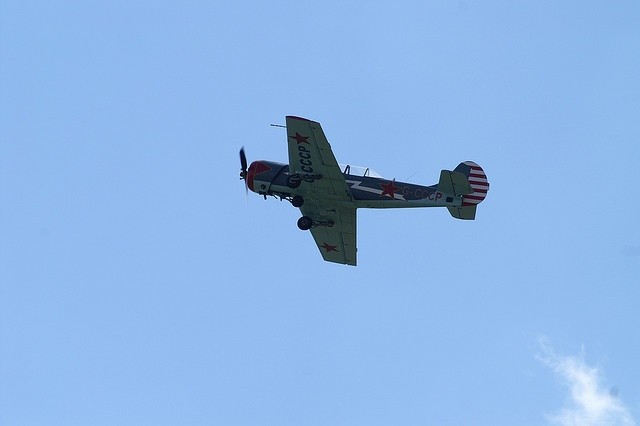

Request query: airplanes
[{"left": 239, "top": 115, "right": 489, "bottom": 267}]
